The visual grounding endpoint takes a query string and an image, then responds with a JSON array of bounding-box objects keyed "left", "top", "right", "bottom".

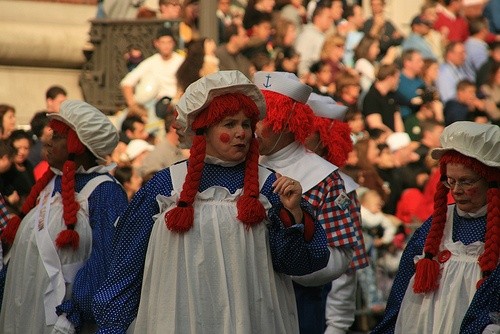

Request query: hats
[
  {"left": 174, "top": 69, "right": 267, "bottom": 131},
  {"left": 253, "top": 70, "right": 313, "bottom": 104},
  {"left": 306, "top": 92, "right": 349, "bottom": 120},
  {"left": 46, "top": 99, "right": 119, "bottom": 163},
  {"left": 430, "top": 120, "right": 500, "bottom": 167}
]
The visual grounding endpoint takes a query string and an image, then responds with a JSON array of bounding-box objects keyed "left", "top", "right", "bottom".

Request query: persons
[
  {"left": 303, "top": 92, "right": 370, "bottom": 334},
  {"left": 90, "top": 69, "right": 331, "bottom": 334},
  {"left": 0, "top": 99, "right": 130, "bottom": 334},
  {"left": 253, "top": 71, "right": 358, "bottom": 334},
  {"left": 0, "top": 0, "right": 500, "bottom": 310},
  {"left": 370, "top": 121, "right": 500, "bottom": 334}
]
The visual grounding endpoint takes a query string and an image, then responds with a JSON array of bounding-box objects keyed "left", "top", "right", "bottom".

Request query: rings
[{"left": 291, "top": 180, "right": 294, "bottom": 185}]
